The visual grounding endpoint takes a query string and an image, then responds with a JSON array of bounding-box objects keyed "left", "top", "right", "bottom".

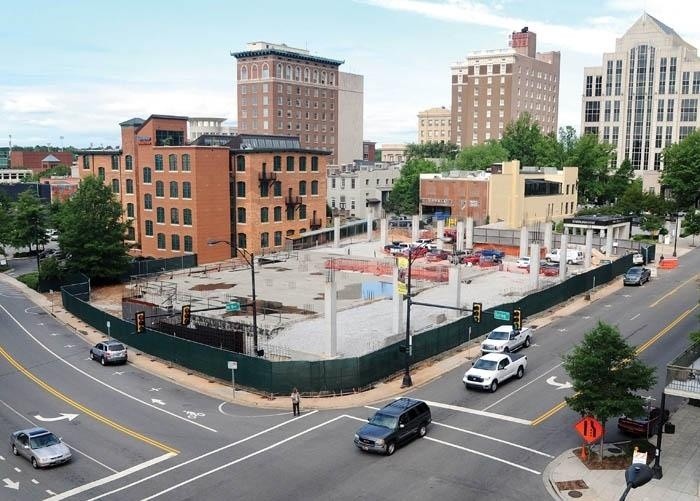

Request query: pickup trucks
[
  {"left": 461, "top": 351, "right": 528, "bottom": 393},
  {"left": 479, "top": 325, "right": 533, "bottom": 355}
]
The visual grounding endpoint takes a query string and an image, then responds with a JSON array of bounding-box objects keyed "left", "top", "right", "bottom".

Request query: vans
[{"left": 545, "top": 249, "right": 584, "bottom": 265}]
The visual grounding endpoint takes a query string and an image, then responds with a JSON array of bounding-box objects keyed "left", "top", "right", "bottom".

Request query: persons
[{"left": 290, "top": 387, "right": 301, "bottom": 416}]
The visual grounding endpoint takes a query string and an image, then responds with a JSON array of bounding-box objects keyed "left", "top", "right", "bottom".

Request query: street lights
[
  {"left": 206, "top": 234, "right": 265, "bottom": 362},
  {"left": 400, "top": 233, "right": 455, "bottom": 390}
]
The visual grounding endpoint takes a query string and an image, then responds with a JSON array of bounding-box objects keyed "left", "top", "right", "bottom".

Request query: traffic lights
[
  {"left": 513, "top": 309, "right": 522, "bottom": 331},
  {"left": 472, "top": 303, "right": 482, "bottom": 324}
]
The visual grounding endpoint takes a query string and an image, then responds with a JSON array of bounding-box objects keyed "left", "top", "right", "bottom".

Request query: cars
[
  {"left": 7, "top": 426, "right": 73, "bottom": 469},
  {"left": 617, "top": 404, "right": 668, "bottom": 439},
  {"left": 383, "top": 237, "right": 532, "bottom": 269},
  {"left": 623, "top": 266, "right": 651, "bottom": 286}
]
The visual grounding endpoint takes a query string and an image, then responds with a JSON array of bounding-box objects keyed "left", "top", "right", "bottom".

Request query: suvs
[
  {"left": 89, "top": 339, "right": 128, "bottom": 366},
  {"left": 352, "top": 396, "right": 432, "bottom": 457}
]
